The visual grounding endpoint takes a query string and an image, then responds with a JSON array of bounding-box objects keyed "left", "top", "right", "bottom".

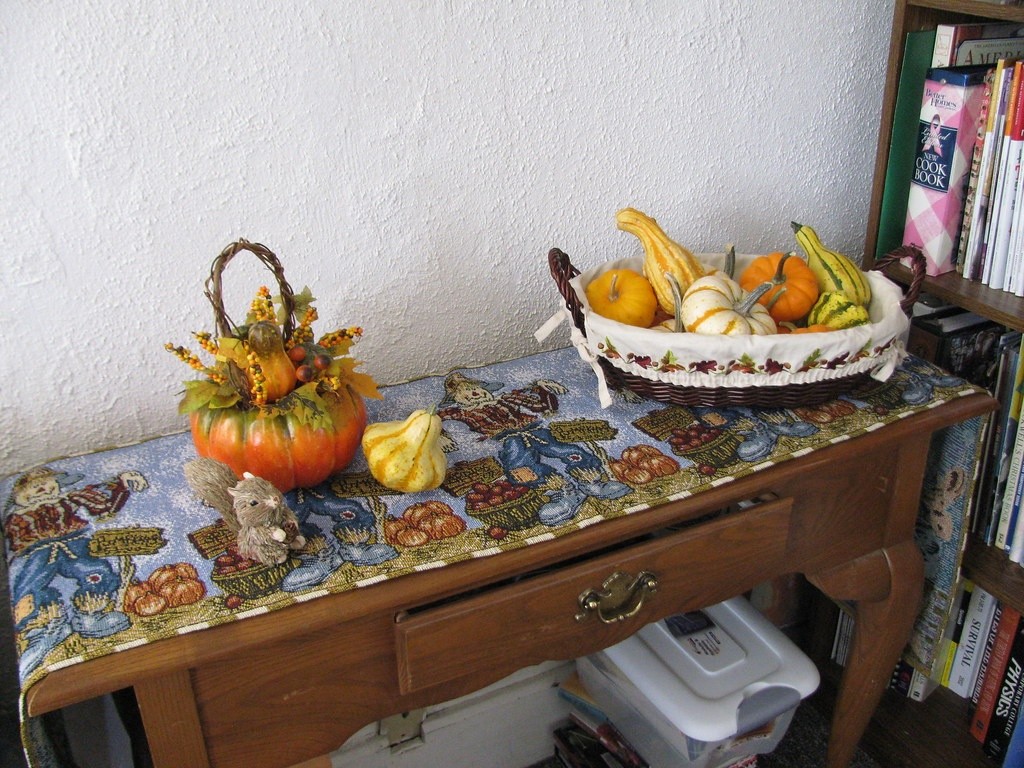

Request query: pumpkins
[
  {"left": 189, "top": 377, "right": 368, "bottom": 497},
  {"left": 362, "top": 403, "right": 448, "bottom": 494},
  {"left": 240, "top": 321, "right": 296, "bottom": 400},
  {"left": 581, "top": 209, "right": 872, "bottom": 338}
]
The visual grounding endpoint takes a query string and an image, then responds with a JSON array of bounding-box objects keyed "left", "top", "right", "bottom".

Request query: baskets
[{"left": 549, "top": 244, "right": 926, "bottom": 408}]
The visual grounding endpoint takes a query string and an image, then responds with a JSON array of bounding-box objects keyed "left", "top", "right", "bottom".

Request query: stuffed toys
[{"left": 183, "top": 458, "right": 305, "bottom": 566}]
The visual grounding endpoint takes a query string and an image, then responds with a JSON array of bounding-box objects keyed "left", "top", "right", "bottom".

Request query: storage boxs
[{"left": 577, "top": 595, "right": 820, "bottom": 768}]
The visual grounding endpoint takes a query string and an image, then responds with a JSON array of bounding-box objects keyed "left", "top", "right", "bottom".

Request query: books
[
  {"left": 811, "top": 574, "right": 1024, "bottom": 768},
  {"left": 906, "top": 295, "right": 1024, "bottom": 568},
  {"left": 549, "top": 670, "right": 758, "bottom": 768},
  {"left": 874, "top": 22, "right": 1024, "bottom": 296}
]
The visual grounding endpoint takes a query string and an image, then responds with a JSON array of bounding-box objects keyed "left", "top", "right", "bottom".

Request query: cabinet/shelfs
[
  {"left": 859, "top": 0, "right": 1024, "bottom": 768},
  {"left": 0, "top": 348, "right": 997, "bottom": 768}
]
